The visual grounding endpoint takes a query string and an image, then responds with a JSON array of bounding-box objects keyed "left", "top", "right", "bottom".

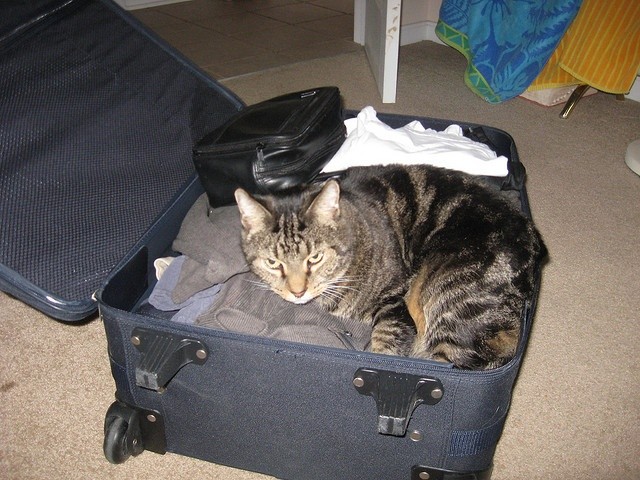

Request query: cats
[{"left": 234, "top": 163, "right": 550, "bottom": 371}]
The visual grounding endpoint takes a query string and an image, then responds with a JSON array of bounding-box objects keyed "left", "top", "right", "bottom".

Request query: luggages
[{"left": 1, "top": 0, "right": 543, "bottom": 480}]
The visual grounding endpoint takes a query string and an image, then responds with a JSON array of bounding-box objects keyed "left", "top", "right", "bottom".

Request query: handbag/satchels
[{"left": 191, "top": 86, "right": 347, "bottom": 209}]
